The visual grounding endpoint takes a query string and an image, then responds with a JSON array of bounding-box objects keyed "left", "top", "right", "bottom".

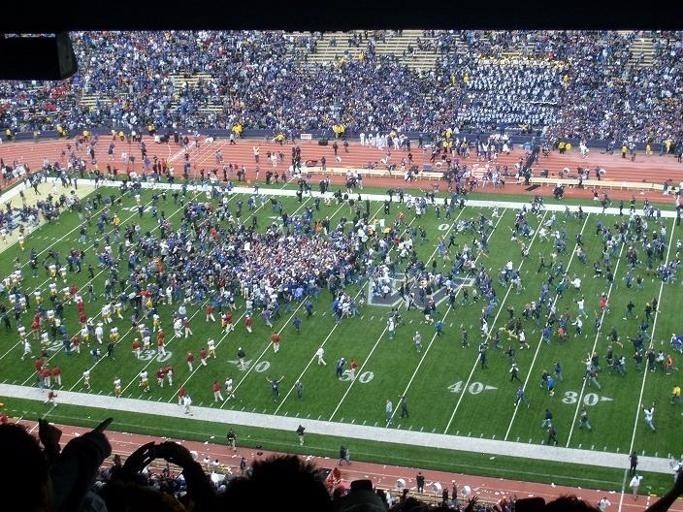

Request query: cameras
[{"left": 149, "top": 445, "right": 171, "bottom": 458}]
